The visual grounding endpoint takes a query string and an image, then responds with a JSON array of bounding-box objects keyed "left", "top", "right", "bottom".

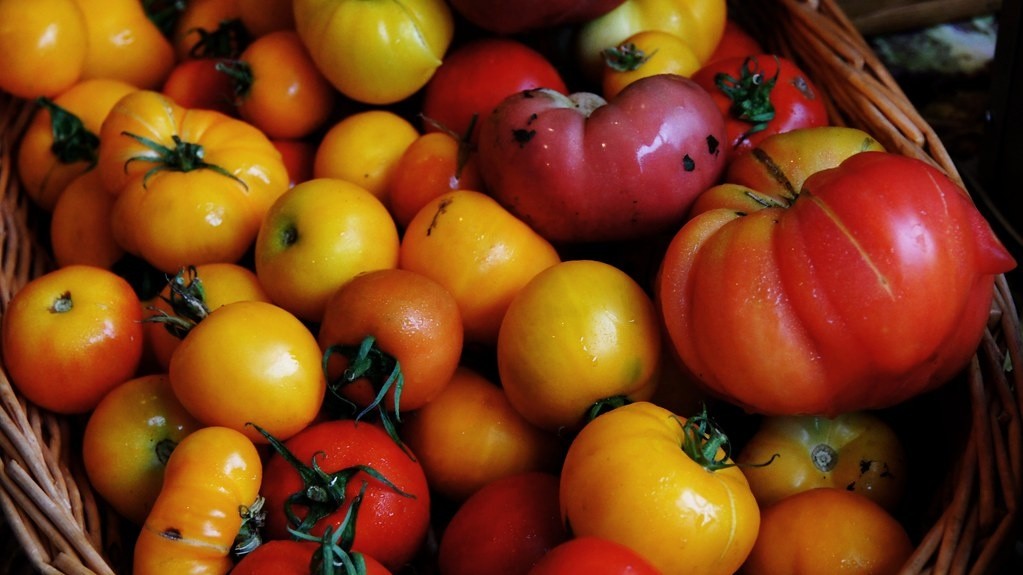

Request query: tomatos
[{"left": 0, "top": 0, "right": 1017, "bottom": 575}]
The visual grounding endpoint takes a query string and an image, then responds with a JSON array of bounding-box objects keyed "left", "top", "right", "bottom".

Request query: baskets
[{"left": 0, "top": 0, "right": 1023, "bottom": 575}]
